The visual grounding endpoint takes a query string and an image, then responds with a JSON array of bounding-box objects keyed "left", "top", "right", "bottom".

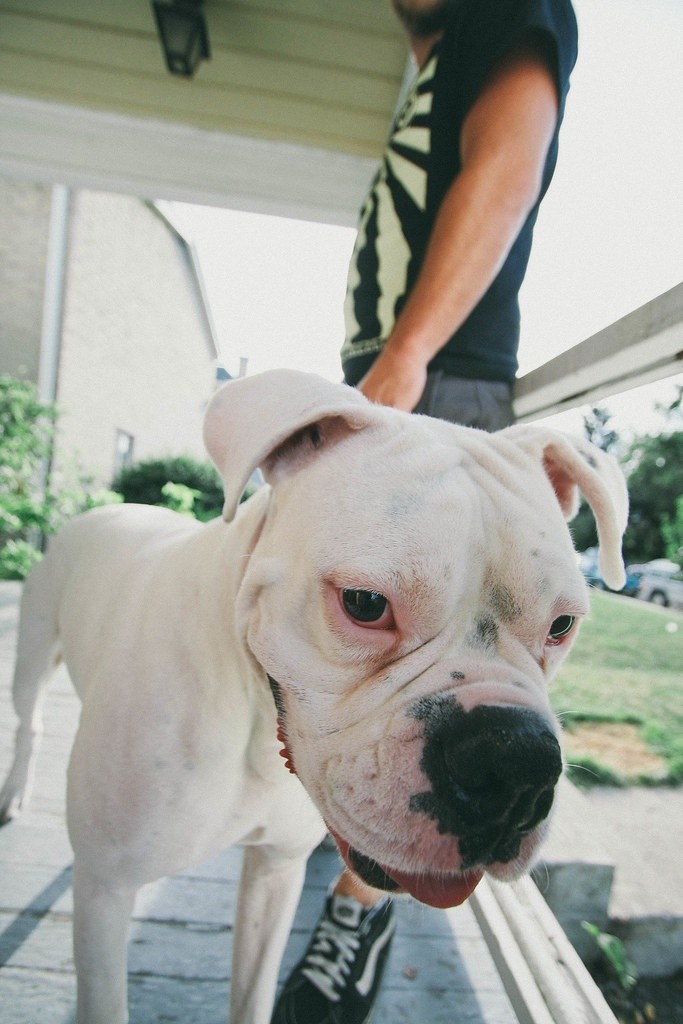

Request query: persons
[{"left": 270, "top": 0, "right": 580, "bottom": 1024}]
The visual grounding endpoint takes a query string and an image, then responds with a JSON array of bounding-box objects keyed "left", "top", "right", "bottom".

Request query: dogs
[{"left": 1, "top": 368, "right": 630, "bottom": 1024}]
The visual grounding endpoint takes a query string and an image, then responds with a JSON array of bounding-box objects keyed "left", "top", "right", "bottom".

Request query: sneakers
[{"left": 270, "top": 894, "right": 397, "bottom": 1024}]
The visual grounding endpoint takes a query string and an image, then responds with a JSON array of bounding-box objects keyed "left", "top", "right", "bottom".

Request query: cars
[{"left": 577, "top": 547, "right": 682, "bottom": 610}]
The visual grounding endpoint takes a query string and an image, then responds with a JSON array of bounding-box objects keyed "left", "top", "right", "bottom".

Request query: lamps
[{"left": 149, "top": 1, "right": 212, "bottom": 78}]
